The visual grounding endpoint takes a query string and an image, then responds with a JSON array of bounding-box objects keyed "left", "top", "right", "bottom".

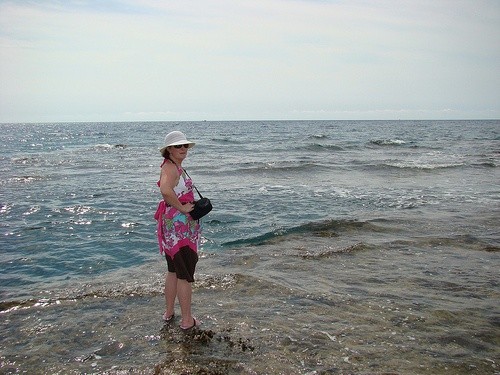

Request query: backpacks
[{"left": 188, "top": 197, "right": 213, "bottom": 221}]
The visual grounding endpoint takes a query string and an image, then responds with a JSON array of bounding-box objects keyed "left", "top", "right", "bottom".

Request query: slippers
[
  {"left": 162, "top": 310, "right": 176, "bottom": 322},
  {"left": 180, "top": 316, "right": 202, "bottom": 331}
]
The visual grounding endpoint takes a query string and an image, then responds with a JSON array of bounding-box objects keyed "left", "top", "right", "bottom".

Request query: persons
[{"left": 153, "top": 131, "right": 203, "bottom": 331}]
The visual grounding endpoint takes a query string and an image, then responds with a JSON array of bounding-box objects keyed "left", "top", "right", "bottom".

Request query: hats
[{"left": 158, "top": 130, "right": 196, "bottom": 154}]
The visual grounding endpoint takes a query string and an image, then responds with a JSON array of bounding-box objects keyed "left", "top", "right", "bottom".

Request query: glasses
[{"left": 172, "top": 144, "right": 191, "bottom": 150}]
